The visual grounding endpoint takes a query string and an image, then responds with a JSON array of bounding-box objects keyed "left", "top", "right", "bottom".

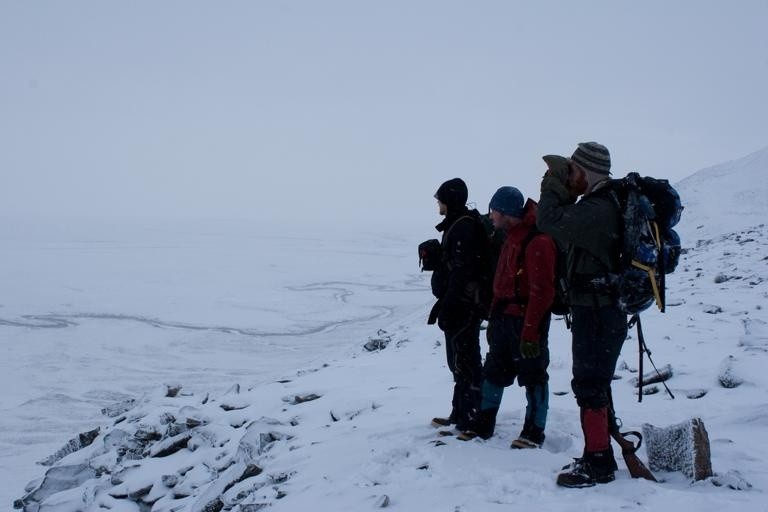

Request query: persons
[
  {"left": 419, "top": 177, "right": 488, "bottom": 434},
  {"left": 457, "top": 186, "right": 558, "bottom": 446},
  {"left": 535, "top": 142, "right": 681, "bottom": 484}
]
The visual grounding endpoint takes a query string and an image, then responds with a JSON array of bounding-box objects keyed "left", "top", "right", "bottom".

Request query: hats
[
  {"left": 488, "top": 186, "right": 524, "bottom": 216},
  {"left": 573, "top": 142, "right": 611, "bottom": 188},
  {"left": 433, "top": 177, "right": 469, "bottom": 216}
]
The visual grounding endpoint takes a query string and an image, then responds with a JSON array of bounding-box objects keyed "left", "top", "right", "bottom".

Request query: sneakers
[
  {"left": 511, "top": 433, "right": 546, "bottom": 449},
  {"left": 456, "top": 427, "right": 495, "bottom": 443},
  {"left": 437, "top": 422, "right": 468, "bottom": 438},
  {"left": 554, "top": 462, "right": 618, "bottom": 488},
  {"left": 561, "top": 444, "right": 619, "bottom": 469},
  {"left": 430, "top": 417, "right": 453, "bottom": 427}
]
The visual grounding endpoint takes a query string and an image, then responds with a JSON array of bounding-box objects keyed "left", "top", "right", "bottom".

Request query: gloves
[{"left": 518, "top": 338, "right": 540, "bottom": 359}]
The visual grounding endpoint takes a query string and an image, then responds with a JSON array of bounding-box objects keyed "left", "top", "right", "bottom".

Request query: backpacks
[
  {"left": 514, "top": 228, "right": 572, "bottom": 316},
  {"left": 442, "top": 214, "right": 508, "bottom": 251},
  {"left": 595, "top": 172, "right": 678, "bottom": 314}
]
